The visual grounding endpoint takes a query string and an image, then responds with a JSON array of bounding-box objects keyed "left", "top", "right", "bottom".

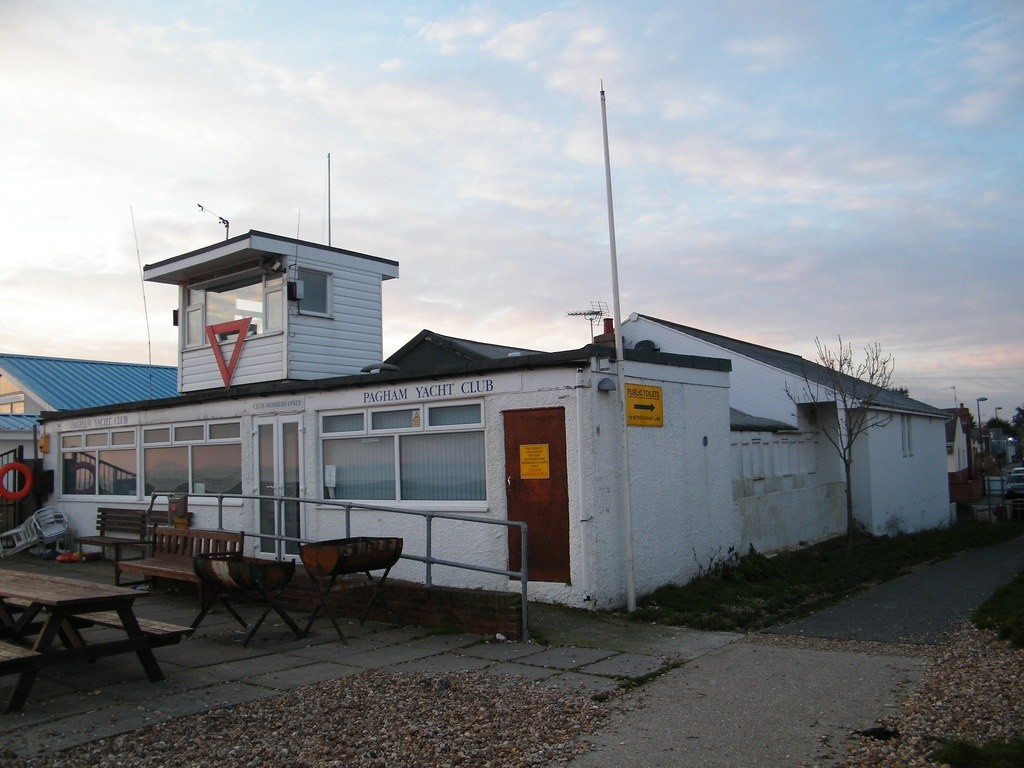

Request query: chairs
[{"left": 1, "top": 506, "right": 72, "bottom": 558}]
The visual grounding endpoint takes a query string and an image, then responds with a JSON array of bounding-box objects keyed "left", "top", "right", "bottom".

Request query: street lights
[
  {"left": 976, "top": 397, "right": 989, "bottom": 477},
  {"left": 995, "top": 405, "right": 1002, "bottom": 462}
]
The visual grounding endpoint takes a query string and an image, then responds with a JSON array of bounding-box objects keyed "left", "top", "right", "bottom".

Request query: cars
[{"left": 1005, "top": 468, "right": 1024, "bottom": 498}]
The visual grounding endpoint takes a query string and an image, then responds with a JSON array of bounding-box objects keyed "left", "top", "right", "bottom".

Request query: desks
[{"left": 0, "top": 569, "right": 166, "bottom": 714}]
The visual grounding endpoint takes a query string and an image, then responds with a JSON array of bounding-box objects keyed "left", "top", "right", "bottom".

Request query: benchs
[
  {"left": 71, "top": 611, "right": 196, "bottom": 682},
  {"left": 113, "top": 523, "right": 245, "bottom": 612},
  {"left": 1, "top": 639, "right": 43, "bottom": 676},
  {"left": 75, "top": 506, "right": 193, "bottom": 571}
]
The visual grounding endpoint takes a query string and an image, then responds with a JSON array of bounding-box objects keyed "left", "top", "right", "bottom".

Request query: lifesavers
[{"left": 0, "top": 462, "right": 32, "bottom": 499}]
